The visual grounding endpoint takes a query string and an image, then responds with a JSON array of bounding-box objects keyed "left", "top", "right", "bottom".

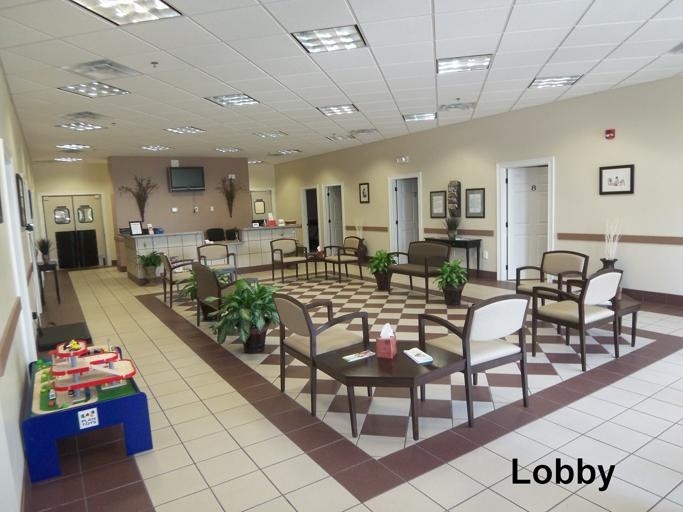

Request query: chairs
[
  {"left": 386, "top": 240, "right": 451, "bottom": 305},
  {"left": 415, "top": 292, "right": 533, "bottom": 430},
  {"left": 270, "top": 236, "right": 364, "bottom": 283},
  {"left": 159, "top": 253, "right": 195, "bottom": 309},
  {"left": 515, "top": 249, "right": 642, "bottom": 371},
  {"left": 271, "top": 290, "right": 373, "bottom": 416},
  {"left": 206, "top": 227, "right": 240, "bottom": 241},
  {"left": 198, "top": 243, "right": 236, "bottom": 277},
  {"left": 191, "top": 260, "right": 259, "bottom": 329}
]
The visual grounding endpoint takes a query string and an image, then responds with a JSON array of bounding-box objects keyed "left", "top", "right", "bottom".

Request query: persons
[{"left": 29, "top": 336, "right": 135, "bottom": 416}]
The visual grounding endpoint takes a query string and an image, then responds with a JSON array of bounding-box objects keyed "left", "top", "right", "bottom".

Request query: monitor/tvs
[
  {"left": 253, "top": 220, "right": 267, "bottom": 226},
  {"left": 167, "top": 167, "right": 206, "bottom": 191}
]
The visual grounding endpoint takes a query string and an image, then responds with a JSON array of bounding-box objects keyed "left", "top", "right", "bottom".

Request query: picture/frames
[
  {"left": 15, "top": 173, "right": 28, "bottom": 227},
  {"left": 599, "top": 164, "right": 634, "bottom": 194},
  {"left": 429, "top": 190, "right": 447, "bottom": 218},
  {"left": 359, "top": 183, "right": 370, "bottom": 204},
  {"left": 129, "top": 220, "right": 143, "bottom": 236},
  {"left": 466, "top": 187, "right": 485, "bottom": 218}
]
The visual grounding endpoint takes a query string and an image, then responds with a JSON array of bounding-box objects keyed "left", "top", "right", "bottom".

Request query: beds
[{"left": 312, "top": 338, "right": 468, "bottom": 440}]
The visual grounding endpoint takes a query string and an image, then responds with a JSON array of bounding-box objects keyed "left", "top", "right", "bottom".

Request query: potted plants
[
  {"left": 136, "top": 250, "right": 180, "bottom": 280},
  {"left": 37, "top": 237, "right": 53, "bottom": 263},
  {"left": 368, "top": 248, "right": 397, "bottom": 291},
  {"left": 432, "top": 256, "right": 467, "bottom": 305},
  {"left": 440, "top": 216, "right": 461, "bottom": 240},
  {"left": 201, "top": 273, "right": 280, "bottom": 354}
]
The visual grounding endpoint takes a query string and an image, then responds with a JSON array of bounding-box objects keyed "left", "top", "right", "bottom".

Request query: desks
[
  {"left": 424, "top": 237, "right": 482, "bottom": 282},
  {"left": 37, "top": 261, "right": 61, "bottom": 305}
]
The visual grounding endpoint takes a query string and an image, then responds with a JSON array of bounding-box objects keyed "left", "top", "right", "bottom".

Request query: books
[
  {"left": 341, "top": 348, "right": 375, "bottom": 363},
  {"left": 402, "top": 347, "right": 433, "bottom": 366}
]
